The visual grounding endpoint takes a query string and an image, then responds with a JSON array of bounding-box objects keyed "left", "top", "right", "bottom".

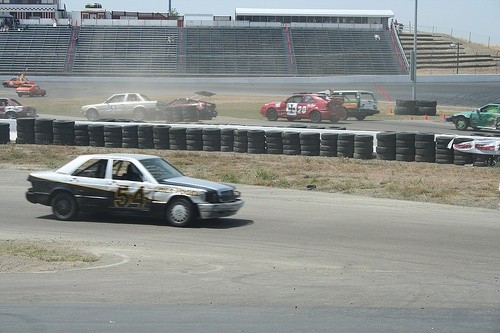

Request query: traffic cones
[
  {"left": 441, "top": 114, "right": 446, "bottom": 121},
  {"left": 423, "top": 114, "right": 428, "bottom": 121},
  {"left": 388, "top": 106, "right": 393, "bottom": 114}
]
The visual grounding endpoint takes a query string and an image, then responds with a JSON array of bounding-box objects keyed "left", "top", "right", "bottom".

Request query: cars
[
  {"left": 260, "top": 92, "right": 347, "bottom": 123},
  {"left": 0, "top": 98, "right": 38, "bottom": 119},
  {"left": 2, "top": 76, "right": 30, "bottom": 88},
  {"left": 16, "top": 83, "right": 46, "bottom": 97},
  {"left": 162, "top": 97, "right": 218, "bottom": 120},
  {"left": 450, "top": 103, "right": 500, "bottom": 133},
  {"left": 25, "top": 154, "right": 244, "bottom": 227},
  {"left": 80, "top": 93, "right": 166, "bottom": 121}
]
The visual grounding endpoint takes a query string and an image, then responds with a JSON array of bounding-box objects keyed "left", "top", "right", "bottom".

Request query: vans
[{"left": 319, "top": 90, "right": 380, "bottom": 120}]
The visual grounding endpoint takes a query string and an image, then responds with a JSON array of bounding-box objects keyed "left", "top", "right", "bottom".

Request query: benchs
[{"left": 0, "top": 24, "right": 405, "bottom": 75}]
